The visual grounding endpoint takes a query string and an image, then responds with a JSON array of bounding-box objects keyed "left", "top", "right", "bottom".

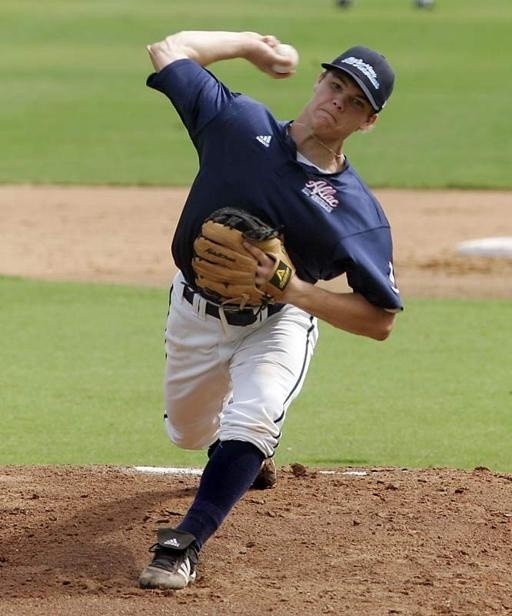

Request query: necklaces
[{"left": 288, "top": 121, "right": 344, "bottom": 159}]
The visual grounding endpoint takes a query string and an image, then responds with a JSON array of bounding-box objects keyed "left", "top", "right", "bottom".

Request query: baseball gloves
[{"left": 192, "top": 207, "right": 297, "bottom": 311}]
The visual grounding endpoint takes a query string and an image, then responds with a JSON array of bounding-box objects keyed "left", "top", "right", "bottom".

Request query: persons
[{"left": 138, "top": 25, "right": 405, "bottom": 590}]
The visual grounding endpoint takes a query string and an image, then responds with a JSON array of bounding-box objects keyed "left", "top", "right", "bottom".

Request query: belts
[{"left": 183, "top": 284, "right": 296, "bottom": 328}]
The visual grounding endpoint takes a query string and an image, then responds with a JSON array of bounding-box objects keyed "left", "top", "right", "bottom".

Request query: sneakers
[
  {"left": 254, "top": 453, "right": 279, "bottom": 491},
  {"left": 137, "top": 526, "right": 203, "bottom": 588}
]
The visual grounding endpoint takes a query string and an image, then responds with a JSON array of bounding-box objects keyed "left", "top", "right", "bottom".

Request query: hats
[{"left": 320, "top": 45, "right": 396, "bottom": 113}]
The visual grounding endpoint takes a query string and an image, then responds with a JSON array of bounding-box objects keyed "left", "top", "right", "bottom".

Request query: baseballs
[{"left": 272, "top": 44, "right": 298, "bottom": 73}]
